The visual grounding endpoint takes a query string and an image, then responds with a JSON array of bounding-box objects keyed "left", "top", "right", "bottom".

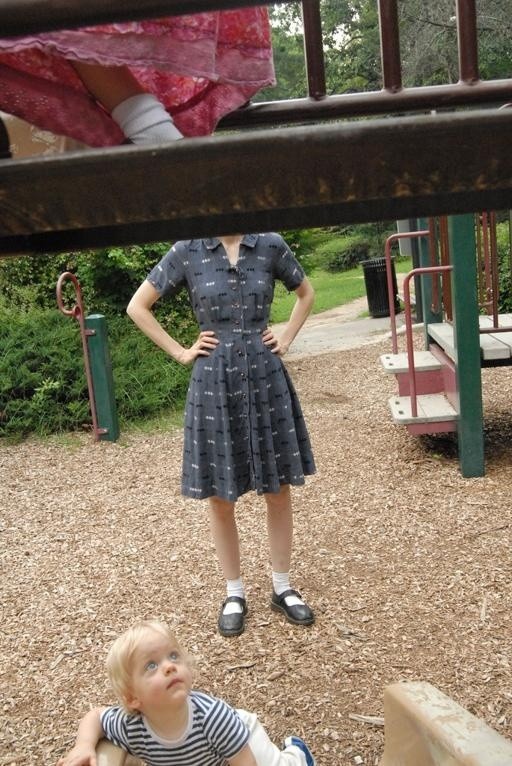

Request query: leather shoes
[
  {"left": 271, "top": 589, "right": 314, "bottom": 625},
  {"left": 219, "top": 596, "right": 247, "bottom": 637}
]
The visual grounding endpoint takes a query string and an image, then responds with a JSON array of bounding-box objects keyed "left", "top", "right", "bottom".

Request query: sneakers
[{"left": 284, "top": 736, "right": 315, "bottom": 766}]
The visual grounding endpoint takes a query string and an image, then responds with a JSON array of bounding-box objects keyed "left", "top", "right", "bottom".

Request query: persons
[
  {"left": 126, "top": 231, "right": 318, "bottom": 637},
  {"left": 55, "top": 620, "right": 317, "bottom": 766},
  {"left": 0, "top": 3, "right": 277, "bottom": 145}
]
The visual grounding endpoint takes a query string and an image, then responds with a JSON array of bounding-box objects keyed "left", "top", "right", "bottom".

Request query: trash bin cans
[{"left": 360, "top": 256, "right": 400, "bottom": 317}]
